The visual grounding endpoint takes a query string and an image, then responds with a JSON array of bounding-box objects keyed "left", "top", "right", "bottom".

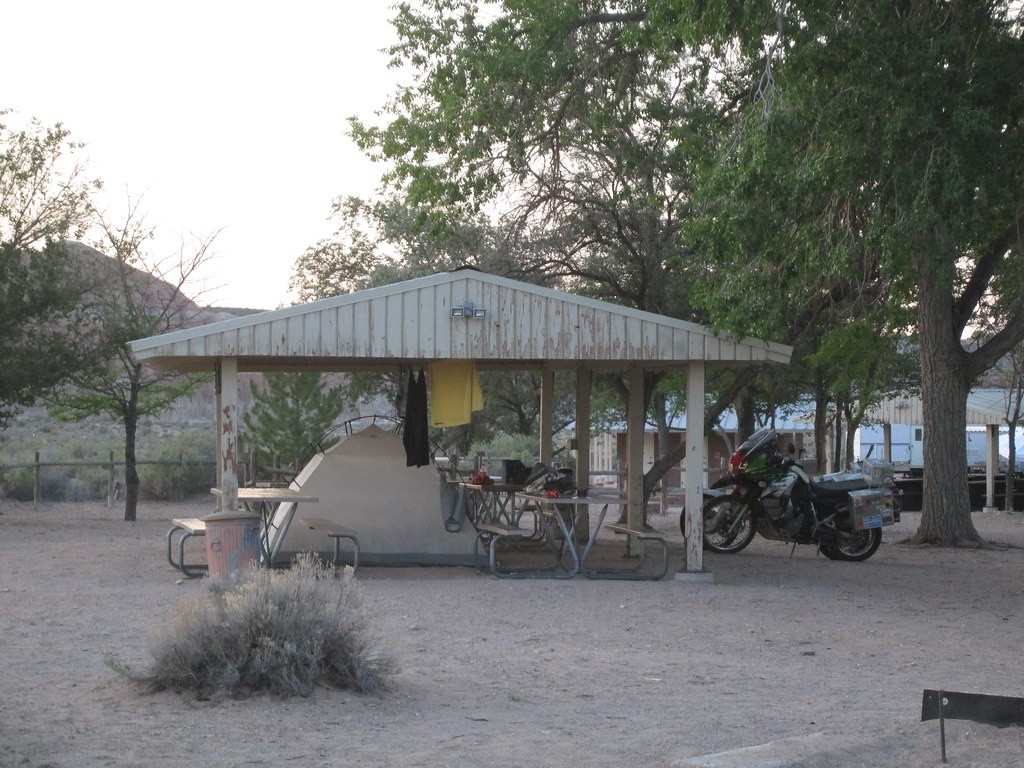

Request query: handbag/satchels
[{"left": 523, "top": 462, "right": 566, "bottom": 495}]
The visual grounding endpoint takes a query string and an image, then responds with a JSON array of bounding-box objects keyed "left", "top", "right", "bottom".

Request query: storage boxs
[
  {"left": 863, "top": 456, "right": 895, "bottom": 485},
  {"left": 851, "top": 488, "right": 894, "bottom": 530}
]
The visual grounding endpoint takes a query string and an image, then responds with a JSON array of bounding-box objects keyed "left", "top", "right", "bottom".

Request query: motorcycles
[{"left": 678, "top": 426, "right": 902, "bottom": 562}]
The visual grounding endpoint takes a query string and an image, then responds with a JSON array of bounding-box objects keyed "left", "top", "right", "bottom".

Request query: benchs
[
  {"left": 165, "top": 516, "right": 207, "bottom": 577},
  {"left": 475, "top": 523, "right": 534, "bottom": 572},
  {"left": 533, "top": 509, "right": 576, "bottom": 540},
  {"left": 604, "top": 520, "right": 669, "bottom": 580},
  {"left": 298, "top": 516, "right": 361, "bottom": 578}
]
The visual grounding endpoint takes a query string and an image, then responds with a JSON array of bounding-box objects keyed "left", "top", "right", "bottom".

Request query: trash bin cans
[{"left": 201, "top": 511, "right": 262, "bottom": 580}]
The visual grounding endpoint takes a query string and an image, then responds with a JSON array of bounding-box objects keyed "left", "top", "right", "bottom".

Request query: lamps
[{"left": 450, "top": 302, "right": 486, "bottom": 318}]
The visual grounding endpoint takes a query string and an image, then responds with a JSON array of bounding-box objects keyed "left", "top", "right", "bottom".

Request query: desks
[
  {"left": 514, "top": 489, "right": 628, "bottom": 579},
  {"left": 210, "top": 485, "right": 320, "bottom": 581},
  {"left": 459, "top": 483, "right": 524, "bottom": 543}
]
